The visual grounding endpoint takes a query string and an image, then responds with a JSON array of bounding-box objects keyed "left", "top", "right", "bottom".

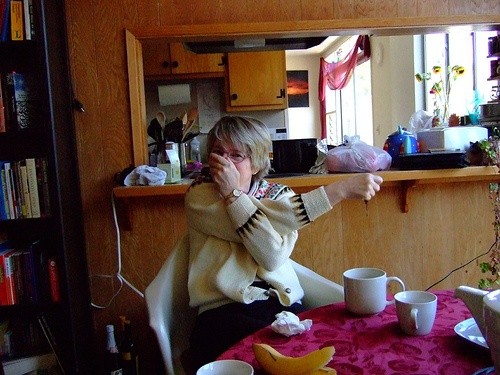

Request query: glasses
[{"left": 212, "top": 150, "right": 248, "bottom": 164}]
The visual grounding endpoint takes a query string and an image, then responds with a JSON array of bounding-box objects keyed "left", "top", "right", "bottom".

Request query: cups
[
  {"left": 393, "top": 291, "right": 438, "bottom": 335},
  {"left": 343, "top": 268, "right": 405, "bottom": 317},
  {"left": 196, "top": 359, "right": 254, "bottom": 375}
]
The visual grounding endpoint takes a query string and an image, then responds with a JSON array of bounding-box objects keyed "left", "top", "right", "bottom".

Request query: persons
[{"left": 186, "top": 118, "right": 383, "bottom": 366}]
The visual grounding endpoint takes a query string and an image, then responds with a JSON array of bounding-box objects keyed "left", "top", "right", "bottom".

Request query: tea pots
[{"left": 455, "top": 285, "right": 500, "bottom": 374}]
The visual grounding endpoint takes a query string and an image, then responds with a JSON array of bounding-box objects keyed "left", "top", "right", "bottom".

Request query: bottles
[
  {"left": 119, "top": 316, "right": 139, "bottom": 375},
  {"left": 191, "top": 137, "right": 201, "bottom": 162},
  {"left": 276, "top": 128, "right": 286, "bottom": 140},
  {"left": 106, "top": 324, "right": 123, "bottom": 375}
]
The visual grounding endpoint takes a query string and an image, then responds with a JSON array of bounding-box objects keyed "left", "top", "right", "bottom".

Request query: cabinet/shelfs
[
  {"left": 143, "top": 43, "right": 289, "bottom": 113},
  {"left": 0, "top": 0, "right": 93, "bottom": 375}
]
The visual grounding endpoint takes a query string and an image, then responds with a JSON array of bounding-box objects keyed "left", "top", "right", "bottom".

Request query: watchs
[{"left": 225, "top": 189, "right": 244, "bottom": 203}]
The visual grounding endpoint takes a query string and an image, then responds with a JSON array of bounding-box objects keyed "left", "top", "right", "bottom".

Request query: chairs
[{"left": 145, "top": 234, "right": 344, "bottom": 375}]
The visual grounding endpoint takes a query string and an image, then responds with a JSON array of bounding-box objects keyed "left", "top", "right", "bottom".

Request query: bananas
[{"left": 252, "top": 343, "right": 338, "bottom": 375}]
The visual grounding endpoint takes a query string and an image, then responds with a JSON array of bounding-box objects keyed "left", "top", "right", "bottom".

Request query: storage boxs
[
  {"left": 396, "top": 150, "right": 466, "bottom": 170},
  {"left": 157, "top": 150, "right": 181, "bottom": 183}
]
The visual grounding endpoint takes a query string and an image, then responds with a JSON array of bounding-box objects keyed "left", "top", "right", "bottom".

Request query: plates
[{"left": 455, "top": 317, "right": 490, "bottom": 350}]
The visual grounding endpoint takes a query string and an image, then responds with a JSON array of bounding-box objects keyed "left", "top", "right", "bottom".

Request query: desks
[{"left": 217, "top": 289, "right": 500, "bottom": 375}]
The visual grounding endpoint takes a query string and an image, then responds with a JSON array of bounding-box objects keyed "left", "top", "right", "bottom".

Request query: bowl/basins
[{"left": 400, "top": 152, "right": 467, "bottom": 170}]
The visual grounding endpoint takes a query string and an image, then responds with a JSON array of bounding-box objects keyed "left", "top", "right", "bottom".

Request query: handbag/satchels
[{"left": 325, "top": 133, "right": 392, "bottom": 172}]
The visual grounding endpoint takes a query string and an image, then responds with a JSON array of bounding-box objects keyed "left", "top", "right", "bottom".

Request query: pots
[{"left": 272, "top": 138, "right": 318, "bottom": 173}]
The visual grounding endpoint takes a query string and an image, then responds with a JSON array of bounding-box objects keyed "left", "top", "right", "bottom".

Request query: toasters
[{"left": 417, "top": 126, "right": 489, "bottom": 150}]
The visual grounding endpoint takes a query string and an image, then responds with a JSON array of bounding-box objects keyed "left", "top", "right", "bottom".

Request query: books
[{"left": 0, "top": 0, "right": 76, "bottom": 375}]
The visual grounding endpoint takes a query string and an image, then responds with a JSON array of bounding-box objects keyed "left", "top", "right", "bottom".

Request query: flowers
[{"left": 415, "top": 65, "right": 465, "bottom": 122}]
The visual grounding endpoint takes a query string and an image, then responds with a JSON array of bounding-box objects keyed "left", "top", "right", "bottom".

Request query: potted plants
[{"left": 147, "top": 110, "right": 202, "bottom": 173}]
